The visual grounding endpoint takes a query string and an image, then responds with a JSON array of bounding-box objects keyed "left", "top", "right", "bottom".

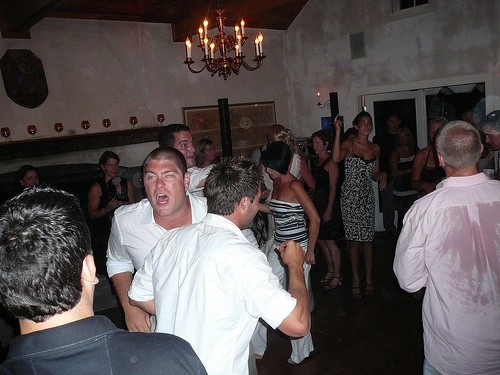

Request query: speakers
[{"left": 321, "top": 116, "right": 343, "bottom": 149}]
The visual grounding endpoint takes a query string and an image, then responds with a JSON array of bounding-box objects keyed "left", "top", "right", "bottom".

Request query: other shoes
[
  {"left": 387, "top": 225, "right": 398, "bottom": 235},
  {"left": 287, "top": 352, "right": 311, "bottom": 364}
]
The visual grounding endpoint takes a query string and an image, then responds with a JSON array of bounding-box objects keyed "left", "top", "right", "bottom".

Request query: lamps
[
  {"left": 316, "top": 91, "right": 331, "bottom": 111},
  {"left": 182, "top": 3, "right": 268, "bottom": 81}
]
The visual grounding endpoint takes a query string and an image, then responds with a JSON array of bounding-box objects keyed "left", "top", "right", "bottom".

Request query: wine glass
[
  {"left": 27, "top": 125, "right": 37, "bottom": 141},
  {"left": 1, "top": 127, "right": 11, "bottom": 144},
  {"left": 81, "top": 120, "right": 90, "bottom": 135},
  {"left": 157, "top": 114, "right": 165, "bottom": 127},
  {"left": 129, "top": 116, "right": 138, "bottom": 130},
  {"left": 54, "top": 122, "right": 63, "bottom": 138},
  {"left": 102, "top": 119, "right": 111, "bottom": 133}
]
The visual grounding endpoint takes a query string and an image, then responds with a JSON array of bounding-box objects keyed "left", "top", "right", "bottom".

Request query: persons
[
  {"left": 250, "top": 112, "right": 387, "bottom": 311},
  {"left": 0, "top": 188, "right": 207, "bottom": 375},
  {"left": 463, "top": 109, "right": 500, "bottom": 180},
  {"left": 88, "top": 124, "right": 311, "bottom": 375},
  {"left": 16, "top": 165, "right": 40, "bottom": 187},
  {"left": 372, "top": 114, "right": 448, "bottom": 237},
  {"left": 393, "top": 120, "right": 500, "bottom": 375}
]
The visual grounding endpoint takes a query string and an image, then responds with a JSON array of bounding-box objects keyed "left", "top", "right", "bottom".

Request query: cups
[{"left": 483, "top": 169, "right": 494, "bottom": 180}]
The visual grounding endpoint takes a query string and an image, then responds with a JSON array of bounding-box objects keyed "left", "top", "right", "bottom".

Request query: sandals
[
  {"left": 351, "top": 282, "right": 362, "bottom": 296},
  {"left": 365, "top": 284, "right": 374, "bottom": 294}
]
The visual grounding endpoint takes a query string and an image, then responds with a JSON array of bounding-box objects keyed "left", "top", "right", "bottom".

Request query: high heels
[
  {"left": 319, "top": 271, "right": 334, "bottom": 283},
  {"left": 324, "top": 274, "right": 343, "bottom": 289}
]
